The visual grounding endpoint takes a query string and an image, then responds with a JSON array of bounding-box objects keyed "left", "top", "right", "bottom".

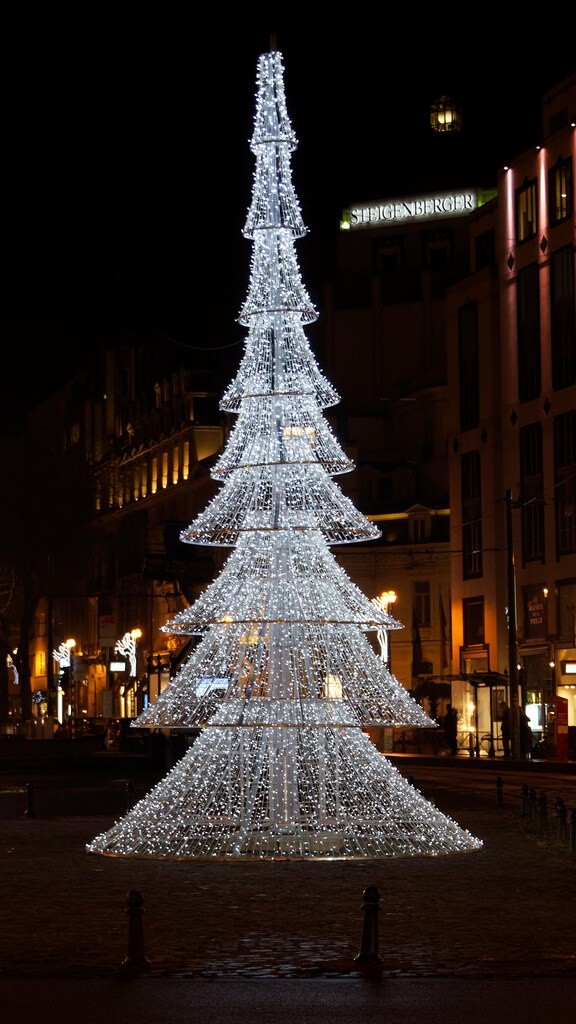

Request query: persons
[
  {"left": 442, "top": 704, "right": 458, "bottom": 755},
  {"left": 499, "top": 701, "right": 533, "bottom": 763}
]
